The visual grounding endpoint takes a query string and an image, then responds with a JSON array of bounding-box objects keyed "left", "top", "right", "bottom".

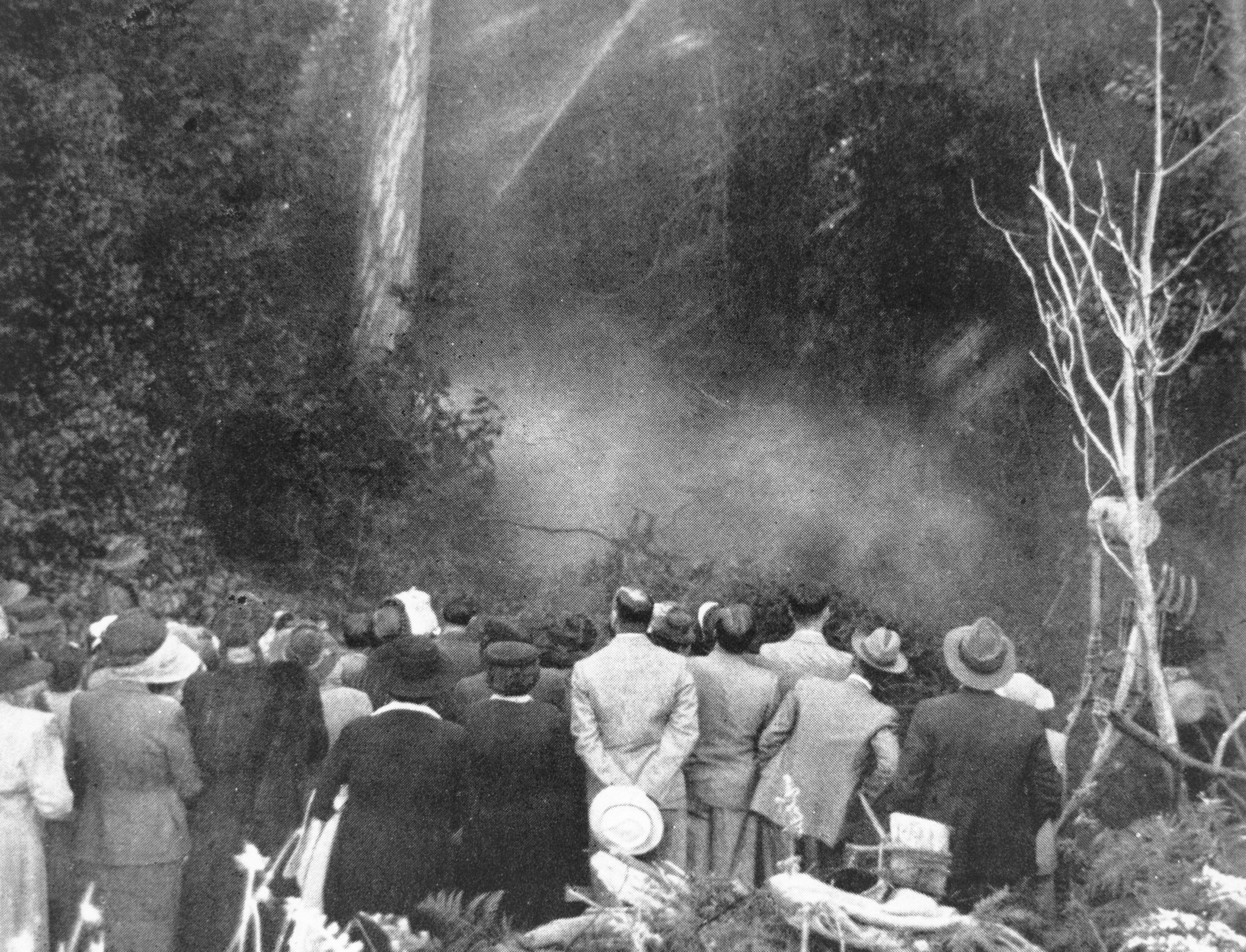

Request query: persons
[{"left": 0, "top": 580, "right": 1065, "bottom": 952}]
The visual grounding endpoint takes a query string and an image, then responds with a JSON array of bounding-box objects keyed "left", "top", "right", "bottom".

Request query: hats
[
  {"left": 88, "top": 610, "right": 202, "bottom": 689},
  {"left": 649, "top": 606, "right": 697, "bottom": 645},
  {"left": 369, "top": 633, "right": 454, "bottom": 700},
  {"left": 588, "top": 786, "right": 663, "bottom": 857},
  {"left": 996, "top": 673, "right": 1054, "bottom": 712},
  {"left": 484, "top": 640, "right": 541, "bottom": 666},
  {"left": 284, "top": 621, "right": 339, "bottom": 681},
  {"left": 0, "top": 638, "right": 53, "bottom": 692},
  {"left": 947, "top": 616, "right": 1016, "bottom": 691},
  {"left": 851, "top": 627, "right": 908, "bottom": 675}
]
